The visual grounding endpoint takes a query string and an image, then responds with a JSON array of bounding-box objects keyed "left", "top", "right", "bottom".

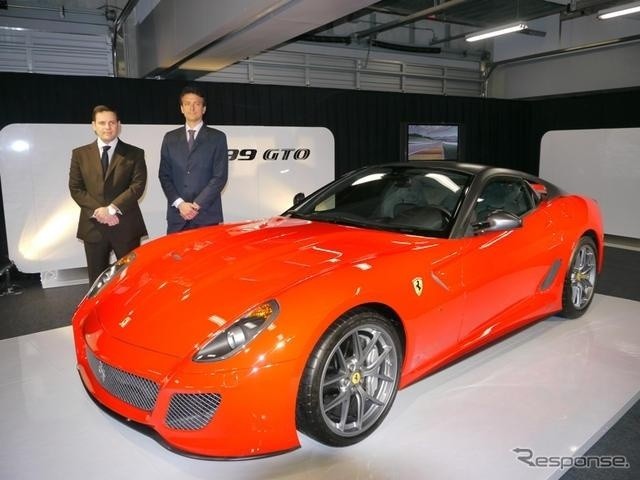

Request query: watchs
[{"left": 108, "top": 205, "right": 118, "bottom": 217}]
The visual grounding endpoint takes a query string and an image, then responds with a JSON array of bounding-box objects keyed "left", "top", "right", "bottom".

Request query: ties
[
  {"left": 101, "top": 145, "right": 110, "bottom": 180},
  {"left": 187, "top": 129, "right": 196, "bottom": 153}
]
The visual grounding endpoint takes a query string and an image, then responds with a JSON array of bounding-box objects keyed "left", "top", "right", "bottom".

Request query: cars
[{"left": 70, "top": 157, "right": 606, "bottom": 465}]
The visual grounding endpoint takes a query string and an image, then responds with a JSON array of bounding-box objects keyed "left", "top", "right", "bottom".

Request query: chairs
[{"left": 369, "top": 179, "right": 522, "bottom": 229}]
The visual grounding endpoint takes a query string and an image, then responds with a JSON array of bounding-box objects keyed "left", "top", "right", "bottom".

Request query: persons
[
  {"left": 69, "top": 103, "right": 148, "bottom": 289},
  {"left": 157, "top": 83, "right": 230, "bottom": 235}
]
